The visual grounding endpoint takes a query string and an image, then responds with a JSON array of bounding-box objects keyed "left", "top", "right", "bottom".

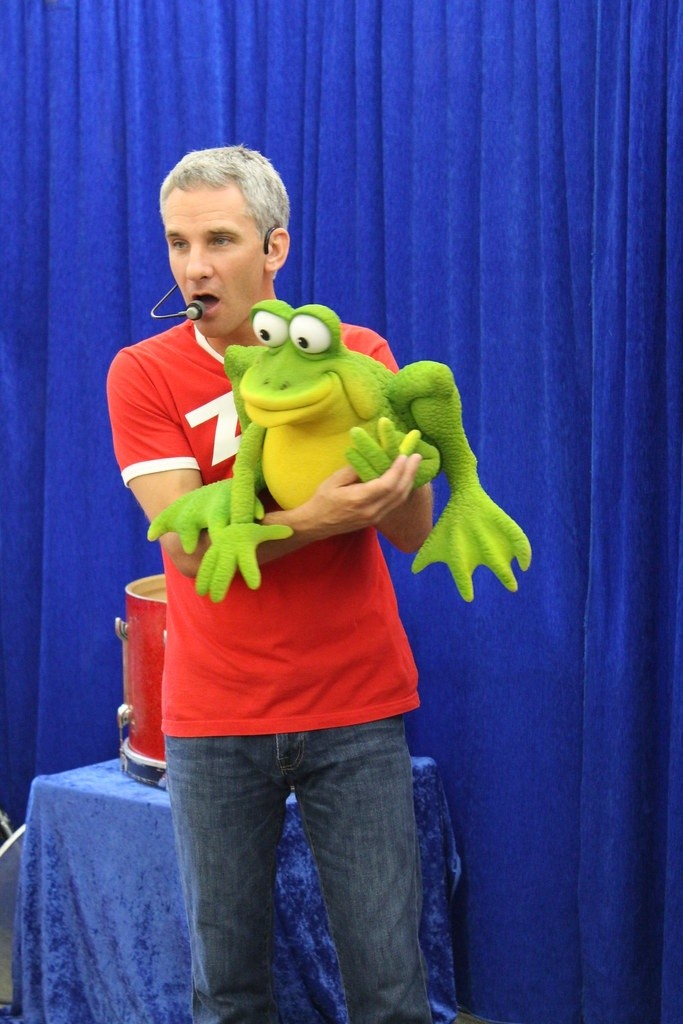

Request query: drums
[{"left": 115, "top": 574, "right": 167, "bottom": 791}]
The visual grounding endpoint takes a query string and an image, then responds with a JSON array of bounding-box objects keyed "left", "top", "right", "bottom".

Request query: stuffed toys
[{"left": 147, "top": 299, "right": 532, "bottom": 602}]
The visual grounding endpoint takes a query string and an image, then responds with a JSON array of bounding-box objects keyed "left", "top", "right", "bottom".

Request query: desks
[{"left": 13, "top": 756, "right": 464, "bottom": 1023}]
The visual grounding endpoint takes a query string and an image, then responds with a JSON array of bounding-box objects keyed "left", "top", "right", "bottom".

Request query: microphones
[{"left": 150, "top": 285, "right": 205, "bottom": 320}]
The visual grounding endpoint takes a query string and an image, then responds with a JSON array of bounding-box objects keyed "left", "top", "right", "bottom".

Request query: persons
[{"left": 105, "top": 146, "right": 432, "bottom": 1024}]
